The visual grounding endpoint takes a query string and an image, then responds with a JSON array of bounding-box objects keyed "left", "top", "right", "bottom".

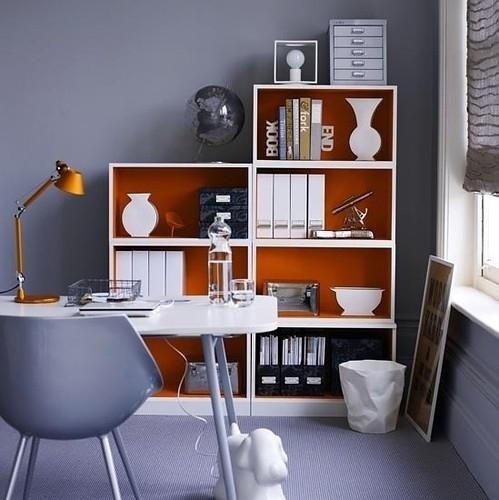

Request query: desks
[{"left": 0, "top": 295, "right": 278, "bottom": 499}]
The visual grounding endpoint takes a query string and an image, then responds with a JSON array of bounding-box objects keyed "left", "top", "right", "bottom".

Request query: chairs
[{"left": 1, "top": 314, "right": 164, "bottom": 500}]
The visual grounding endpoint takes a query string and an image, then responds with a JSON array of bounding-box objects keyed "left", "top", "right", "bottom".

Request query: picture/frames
[{"left": 403, "top": 254, "right": 456, "bottom": 444}]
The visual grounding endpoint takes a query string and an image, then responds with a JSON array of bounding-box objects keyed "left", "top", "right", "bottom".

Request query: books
[
  {"left": 279, "top": 97, "right": 324, "bottom": 161},
  {"left": 259, "top": 333, "right": 327, "bottom": 386}
]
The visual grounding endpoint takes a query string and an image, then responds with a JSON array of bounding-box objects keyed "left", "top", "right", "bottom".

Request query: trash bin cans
[{"left": 339, "top": 360, "right": 407, "bottom": 434}]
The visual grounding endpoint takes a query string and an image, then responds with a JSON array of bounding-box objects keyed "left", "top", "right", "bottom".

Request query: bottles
[{"left": 207, "top": 216, "right": 233, "bottom": 305}]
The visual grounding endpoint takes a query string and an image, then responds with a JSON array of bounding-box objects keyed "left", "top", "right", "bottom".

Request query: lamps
[{"left": 11, "top": 159, "right": 86, "bottom": 304}]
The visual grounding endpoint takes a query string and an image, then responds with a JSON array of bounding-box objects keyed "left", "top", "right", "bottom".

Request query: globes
[{"left": 184, "top": 84, "right": 246, "bottom": 163}]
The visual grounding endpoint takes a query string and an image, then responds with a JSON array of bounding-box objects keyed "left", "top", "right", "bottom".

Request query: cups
[{"left": 230, "top": 278, "right": 255, "bottom": 308}]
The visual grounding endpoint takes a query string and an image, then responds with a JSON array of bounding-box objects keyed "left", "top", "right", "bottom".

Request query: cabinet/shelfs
[
  {"left": 326, "top": 19, "right": 389, "bottom": 86},
  {"left": 251, "top": 84, "right": 399, "bottom": 422},
  {"left": 108, "top": 163, "right": 254, "bottom": 416}
]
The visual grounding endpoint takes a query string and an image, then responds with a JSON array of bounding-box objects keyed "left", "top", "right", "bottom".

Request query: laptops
[{"left": 78, "top": 300, "right": 160, "bottom": 318}]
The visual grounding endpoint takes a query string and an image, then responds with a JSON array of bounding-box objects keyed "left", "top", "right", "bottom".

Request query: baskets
[{"left": 64, "top": 277, "right": 142, "bottom": 304}]
